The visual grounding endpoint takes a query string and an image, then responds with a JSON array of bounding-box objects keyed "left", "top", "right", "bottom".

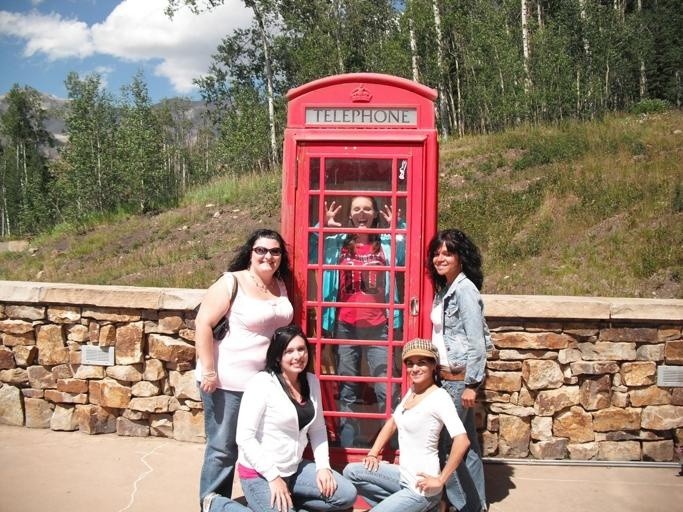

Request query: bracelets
[
  {"left": 367, "top": 454, "right": 377, "bottom": 459},
  {"left": 205, "top": 371, "right": 217, "bottom": 381}
]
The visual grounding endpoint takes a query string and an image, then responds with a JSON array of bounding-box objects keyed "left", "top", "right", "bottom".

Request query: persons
[
  {"left": 429, "top": 228, "right": 493, "bottom": 512},
  {"left": 339, "top": 337, "right": 472, "bottom": 511},
  {"left": 307, "top": 194, "right": 407, "bottom": 451},
  {"left": 192, "top": 229, "right": 296, "bottom": 510},
  {"left": 204, "top": 324, "right": 357, "bottom": 511}
]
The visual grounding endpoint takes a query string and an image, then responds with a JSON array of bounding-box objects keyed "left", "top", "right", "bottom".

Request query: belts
[{"left": 438, "top": 371, "right": 467, "bottom": 381}]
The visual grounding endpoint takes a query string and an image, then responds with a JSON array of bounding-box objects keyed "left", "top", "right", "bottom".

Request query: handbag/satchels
[{"left": 192, "top": 302, "right": 229, "bottom": 340}]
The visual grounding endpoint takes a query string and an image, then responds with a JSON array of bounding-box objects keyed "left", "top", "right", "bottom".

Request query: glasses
[
  {"left": 252, "top": 247, "right": 281, "bottom": 256},
  {"left": 274, "top": 325, "right": 299, "bottom": 341}
]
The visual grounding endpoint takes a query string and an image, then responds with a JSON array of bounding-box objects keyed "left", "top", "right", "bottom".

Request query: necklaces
[
  {"left": 398, "top": 391, "right": 426, "bottom": 413},
  {"left": 249, "top": 273, "right": 276, "bottom": 294}
]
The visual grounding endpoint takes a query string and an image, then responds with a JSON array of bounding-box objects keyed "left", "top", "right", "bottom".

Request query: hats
[{"left": 402, "top": 338, "right": 440, "bottom": 366}]
